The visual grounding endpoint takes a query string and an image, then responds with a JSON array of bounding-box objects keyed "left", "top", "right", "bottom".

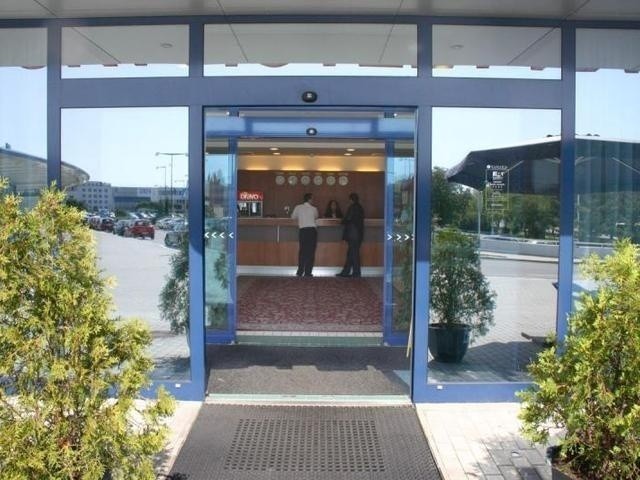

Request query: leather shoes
[{"left": 296, "top": 275, "right": 319, "bottom": 281}]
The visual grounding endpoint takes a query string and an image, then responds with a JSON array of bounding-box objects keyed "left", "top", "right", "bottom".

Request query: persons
[
  {"left": 323, "top": 200, "right": 343, "bottom": 218},
  {"left": 336, "top": 193, "right": 364, "bottom": 277},
  {"left": 290, "top": 193, "right": 319, "bottom": 277}
]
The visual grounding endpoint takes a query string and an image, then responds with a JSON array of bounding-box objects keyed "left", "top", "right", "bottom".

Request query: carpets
[{"left": 234, "top": 275, "right": 411, "bottom": 332}]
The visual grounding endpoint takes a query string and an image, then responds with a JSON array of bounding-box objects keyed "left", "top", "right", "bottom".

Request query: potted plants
[
  {"left": 514, "top": 228, "right": 639, "bottom": 477},
  {"left": 429, "top": 226, "right": 496, "bottom": 364}
]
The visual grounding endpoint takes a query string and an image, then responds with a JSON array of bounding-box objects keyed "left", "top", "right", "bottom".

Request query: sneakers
[{"left": 336, "top": 273, "right": 346, "bottom": 276}]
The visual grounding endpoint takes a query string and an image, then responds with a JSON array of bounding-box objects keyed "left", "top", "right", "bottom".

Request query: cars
[{"left": 81, "top": 208, "right": 232, "bottom": 247}]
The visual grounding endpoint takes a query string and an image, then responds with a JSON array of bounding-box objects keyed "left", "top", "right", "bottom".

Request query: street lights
[
  {"left": 155, "top": 151, "right": 188, "bottom": 215},
  {"left": 546, "top": 133, "right": 600, "bottom": 139}
]
